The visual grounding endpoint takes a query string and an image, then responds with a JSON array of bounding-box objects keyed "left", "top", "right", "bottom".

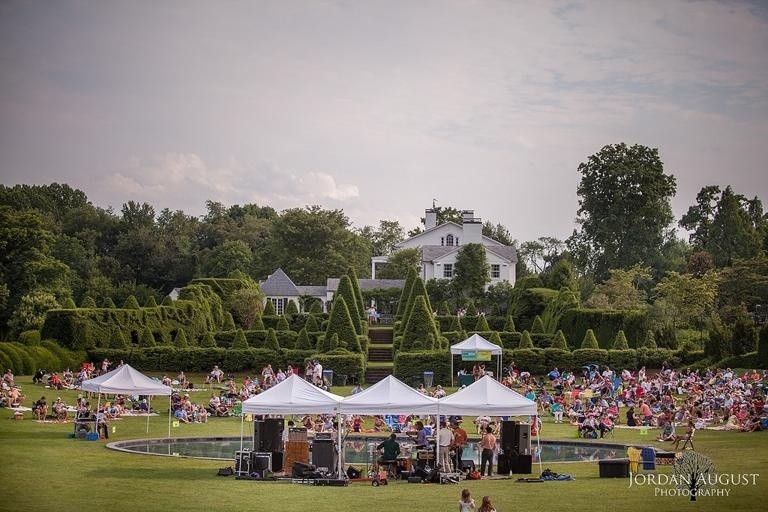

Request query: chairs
[{"left": 672, "top": 428, "right": 695, "bottom": 450}]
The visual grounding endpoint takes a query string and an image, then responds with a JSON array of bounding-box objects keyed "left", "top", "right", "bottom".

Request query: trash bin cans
[
  {"left": 642, "top": 448, "right": 656, "bottom": 470},
  {"left": 323, "top": 369, "right": 333, "bottom": 387},
  {"left": 424, "top": 372, "right": 434, "bottom": 388},
  {"left": 413, "top": 376, "right": 423, "bottom": 388},
  {"left": 337, "top": 375, "right": 347, "bottom": 386}
]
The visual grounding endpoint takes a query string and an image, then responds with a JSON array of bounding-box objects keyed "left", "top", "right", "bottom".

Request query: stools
[{"left": 382, "top": 459, "right": 397, "bottom": 480}]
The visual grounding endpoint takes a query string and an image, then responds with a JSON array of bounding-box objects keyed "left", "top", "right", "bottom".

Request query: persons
[
  {"left": 366, "top": 301, "right": 487, "bottom": 327},
  {"left": 478, "top": 495, "right": 495, "bottom": 512},
  {"left": 458, "top": 488, "right": 477, "bottom": 512},
  {"left": 0, "top": 355, "right": 766, "bottom": 476}
]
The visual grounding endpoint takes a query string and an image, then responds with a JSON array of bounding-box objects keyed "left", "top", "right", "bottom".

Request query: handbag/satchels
[{"left": 217, "top": 466, "right": 233, "bottom": 476}]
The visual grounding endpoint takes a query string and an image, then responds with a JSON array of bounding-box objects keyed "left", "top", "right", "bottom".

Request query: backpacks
[
  {"left": 423, "top": 463, "right": 442, "bottom": 482},
  {"left": 542, "top": 468, "right": 556, "bottom": 478},
  {"left": 292, "top": 459, "right": 318, "bottom": 478}
]
